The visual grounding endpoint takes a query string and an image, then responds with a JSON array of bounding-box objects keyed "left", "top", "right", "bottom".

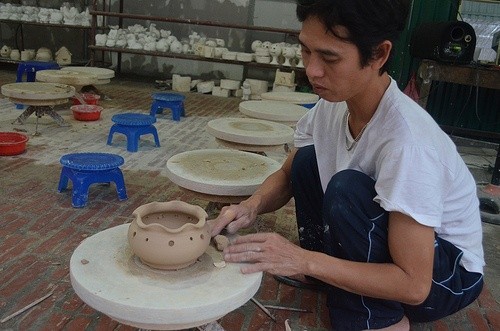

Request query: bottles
[{"left": 11, "top": 49, "right": 20, "bottom": 60}]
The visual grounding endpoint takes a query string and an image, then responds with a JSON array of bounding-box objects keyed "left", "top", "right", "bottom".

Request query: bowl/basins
[
  {"left": 70, "top": 105, "right": 103, "bottom": 121},
  {"left": 0, "top": 131, "right": 29, "bottom": 156},
  {"left": 71, "top": 94, "right": 101, "bottom": 106}
]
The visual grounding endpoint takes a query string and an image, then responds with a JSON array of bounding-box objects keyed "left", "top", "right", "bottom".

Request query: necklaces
[{"left": 344, "top": 110, "right": 367, "bottom": 152}]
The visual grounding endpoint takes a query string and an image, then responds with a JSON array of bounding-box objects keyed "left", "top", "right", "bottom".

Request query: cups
[{"left": 20, "top": 48, "right": 35, "bottom": 61}]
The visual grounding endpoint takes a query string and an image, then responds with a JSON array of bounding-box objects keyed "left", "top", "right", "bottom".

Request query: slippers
[{"left": 273, "top": 273, "right": 335, "bottom": 294}]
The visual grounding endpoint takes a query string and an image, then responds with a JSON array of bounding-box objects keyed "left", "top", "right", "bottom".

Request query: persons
[{"left": 206, "top": 0, "right": 487, "bottom": 330}]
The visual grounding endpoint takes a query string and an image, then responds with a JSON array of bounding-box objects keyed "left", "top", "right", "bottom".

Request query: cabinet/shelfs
[
  {"left": 0, "top": 0, "right": 107, "bottom": 68},
  {"left": 87, "top": 10, "right": 313, "bottom": 94}
]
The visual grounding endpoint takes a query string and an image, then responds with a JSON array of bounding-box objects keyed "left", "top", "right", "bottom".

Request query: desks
[{"left": 418, "top": 56, "right": 500, "bottom": 110}]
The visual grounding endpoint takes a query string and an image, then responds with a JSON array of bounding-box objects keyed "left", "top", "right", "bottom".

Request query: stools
[
  {"left": 107, "top": 113, "right": 160, "bottom": 152},
  {"left": 58, "top": 152, "right": 128, "bottom": 208},
  {"left": 150, "top": 93, "right": 186, "bottom": 121}
]
